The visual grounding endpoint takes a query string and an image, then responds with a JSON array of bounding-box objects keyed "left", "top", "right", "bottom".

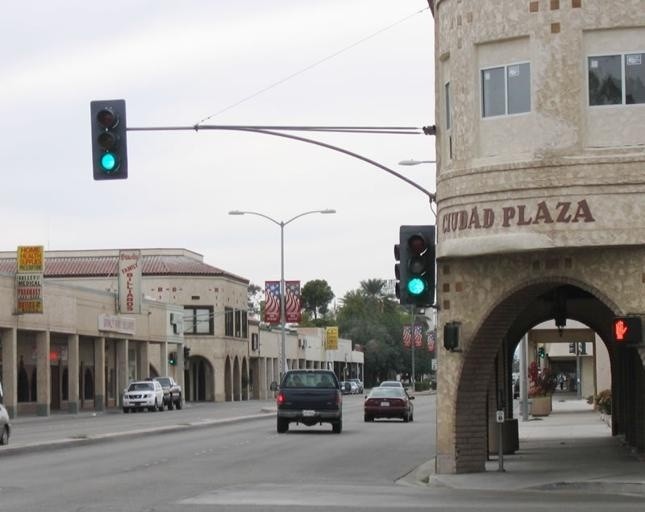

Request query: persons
[{"left": 559, "top": 373, "right": 564, "bottom": 390}]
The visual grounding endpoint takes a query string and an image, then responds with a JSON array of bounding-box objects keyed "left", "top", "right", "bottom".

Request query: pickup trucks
[{"left": 270, "top": 369, "right": 350, "bottom": 433}]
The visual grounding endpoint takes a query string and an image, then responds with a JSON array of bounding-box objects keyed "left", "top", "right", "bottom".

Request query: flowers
[{"left": 527, "top": 361, "right": 551, "bottom": 397}]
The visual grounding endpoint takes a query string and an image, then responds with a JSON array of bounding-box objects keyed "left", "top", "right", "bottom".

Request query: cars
[
  {"left": 363, "top": 381, "right": 414, "bottom": 423},
  {"left": 122, "top": 376, "right": 183, "bottom": 413},
  {"left": 341, "top": 377, "right": 364, "bottom": 395}
]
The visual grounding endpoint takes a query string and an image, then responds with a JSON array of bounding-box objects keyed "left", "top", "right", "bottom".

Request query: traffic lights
[
  {"left": 394, "top": 225, "right": 435, "bottom": 306},
  {"left": 612, "top": 317, "right": 642, "bottom": 345},
  {"left": 168, "top": 352, "right": 176, "bottom": 366},
  {"left": 184, "top": 345, "right": 191, "bottom": 360},
  {"left": 90, "top": 99, "right": 128, "bottom": 180}
]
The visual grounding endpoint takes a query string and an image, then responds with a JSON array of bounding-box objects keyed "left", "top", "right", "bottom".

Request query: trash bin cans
[{"left": 487, "top": 419, "right": 519, "bottom": 454}]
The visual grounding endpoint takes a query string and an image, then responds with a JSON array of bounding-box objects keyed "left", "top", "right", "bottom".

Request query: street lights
[
  {"left": 228, "top": 209, "right": 336, "bottom": 371},
  {"left": 398, "top": 160, "right": 436, "bottom": 166}
]
